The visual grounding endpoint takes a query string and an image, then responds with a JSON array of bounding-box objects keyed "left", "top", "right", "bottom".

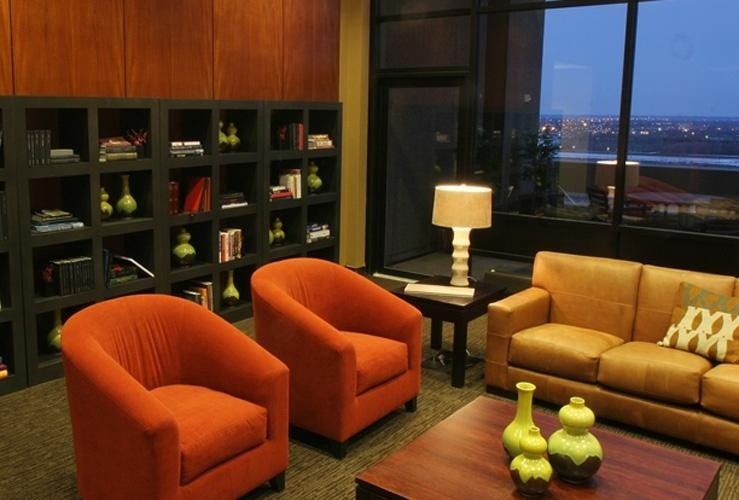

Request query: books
[
  {"left": 198, "top": 282, "right": 213, "bottom": 312},
  {"left": 192, "top": 287, "right": 209, "bottom": 309},
  {"left": 217, "top": 192, "right": 248, "bottom": 210},
  {"left": 268, "top": 167, "right": 301, "bottom": 202},
  {"left": 172, "top": 181, "right": 179, "bottom": 214},
  {"left": 99, "top": 135, "right": 136, "bottom": 164},
  {"left": 371, "top": 271, "right": 419, "bottom": 294},
  {"left": 168, "top": 140, "right": 204, "bottom": 158},
  {"left": 274, "top": 122, "right": 298, "bottom": 152},
  {"left": 102, "top": 248, "right": 139, "bottom": 289},
  {"left": 306, "top": 223, "right": 332, "bottom": 244},
  {"left": 295, "top": 124, "right": 304, "bottom": 152},
  {"left": 25, "top": 129, "right": 95, "bottom": 298},
  {"left": 218, "top": 227, "right": 243, "bottom": 263},
  {"left": 307, "top": 134, "right": 334, "bottom": 151},
  {"left": 168, "top": 183, "right": 174, "bottom": 215},
  {"left": 404, "top": 282, "right": 476, "bottom": 299},
  {"left": 183, "top": 290, "right": 201, "bottom": 305},
  {"left": 182, "top": 176, "right": 211, "bottom": 212}
]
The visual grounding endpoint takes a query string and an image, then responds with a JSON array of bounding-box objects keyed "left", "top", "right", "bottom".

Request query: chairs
[
  {"left": 250, "top": 257, "right": 423, "bottom": 460},
  {"left": 61, "top": 293, "right": 291, "bottom": 500}
]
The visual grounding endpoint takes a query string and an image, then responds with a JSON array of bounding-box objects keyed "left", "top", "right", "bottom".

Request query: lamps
[{"left": 432, "top": 184, "right": 492, "bottom": 287}]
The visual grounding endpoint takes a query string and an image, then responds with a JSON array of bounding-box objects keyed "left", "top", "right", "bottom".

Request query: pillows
[{"left": 657, "top": 281, "right": 739, "bottom": 364}]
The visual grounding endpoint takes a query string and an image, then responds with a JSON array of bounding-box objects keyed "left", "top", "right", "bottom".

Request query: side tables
[{"left": 389, "top": 274, "right": 508, "bottom": 388}]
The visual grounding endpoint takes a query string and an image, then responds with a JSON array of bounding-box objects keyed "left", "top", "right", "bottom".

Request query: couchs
[{"left": 485, "top": 251, "right": 739, "bottom": 463}]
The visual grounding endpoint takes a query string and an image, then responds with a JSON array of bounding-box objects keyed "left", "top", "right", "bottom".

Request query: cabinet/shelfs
[{"left": 0, "top": 0, "right": 343, "bottom": 397}]
